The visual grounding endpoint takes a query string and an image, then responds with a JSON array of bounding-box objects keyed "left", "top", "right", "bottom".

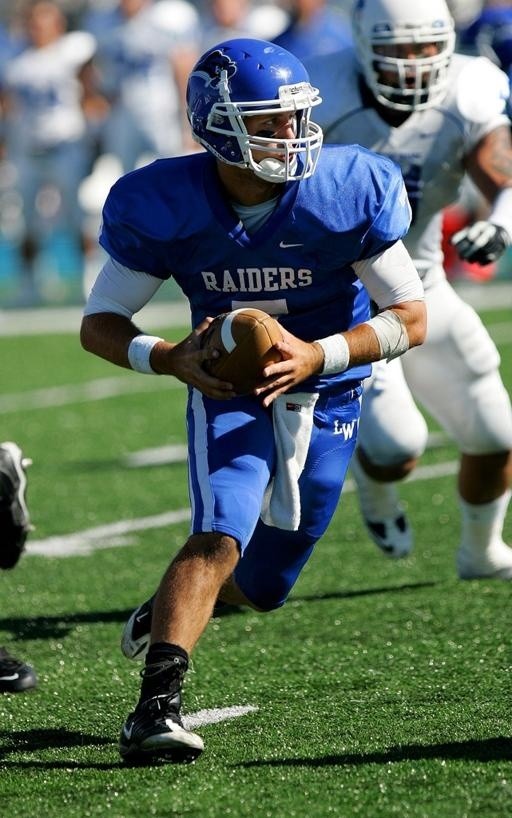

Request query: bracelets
[
  {"left": 128, "top": 334, "right": 165, "bottom": 375},
  {"left": 313, "top": 333, "right": 349, "bottom": 376}
]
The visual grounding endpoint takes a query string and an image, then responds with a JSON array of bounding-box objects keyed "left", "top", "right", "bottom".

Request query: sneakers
[
  {"left": 351, "top": 452, "right": 413, "bottom": 558},
  {"left": 457, "top": 543, "right": 512, "bottom": 582},
  {"left": 0, "top": 439, "right": 31, "bottom": 569},
  {"left": 0, "top": 646, "right": 36, "bottom": 693},
  {"left": 118, "top": 692, "right": 204, "bottom": 764},
  {"left": 120, "top": 595, "right": 154, "bottom": 661}
]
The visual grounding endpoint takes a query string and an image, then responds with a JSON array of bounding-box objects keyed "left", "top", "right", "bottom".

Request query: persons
[
  {"left": 0, "top": 441, "right": 36, "bottom": 693},
  {"left": 80, "top": 37, "right": 428, "bottom": 758},
  {"left": 303, "top": 0, "right": 512, "bottom": 580}
]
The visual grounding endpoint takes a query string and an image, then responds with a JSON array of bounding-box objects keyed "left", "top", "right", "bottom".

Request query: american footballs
[{"left": 199, "top": 307, "right": 283, "bottom": 398}]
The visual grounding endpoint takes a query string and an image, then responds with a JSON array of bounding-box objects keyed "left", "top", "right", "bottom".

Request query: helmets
[
  {"left": 184, "top": 38, "right": 322, "bottom": 162},
  {"left": 351, "top": 0, "right": 456, "bottom": 72}
]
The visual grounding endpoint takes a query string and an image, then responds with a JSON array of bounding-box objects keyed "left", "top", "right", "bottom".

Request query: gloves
[{"left": 448, "top": 220, "right": 511, "bottom": 266}]
